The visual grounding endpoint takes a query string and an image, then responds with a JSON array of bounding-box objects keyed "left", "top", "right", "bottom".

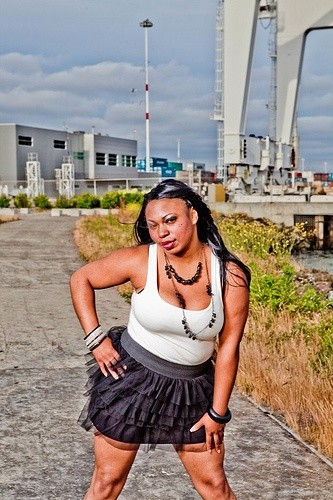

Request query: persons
[{"left": 69, "top": 178, "right": 251, "bottom": 500}]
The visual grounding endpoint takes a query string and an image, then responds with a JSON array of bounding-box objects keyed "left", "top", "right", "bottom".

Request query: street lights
[{"left": 140, "top": 20, "right": 153, "bottom": 172}]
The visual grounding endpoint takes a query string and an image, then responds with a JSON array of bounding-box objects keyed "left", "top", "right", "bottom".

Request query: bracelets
[
  {"left": 82, "top": 324, "right": 108, "bottom": 351},
  {"left": 206, "top": 406, "right": 233, "bottom": 424}
]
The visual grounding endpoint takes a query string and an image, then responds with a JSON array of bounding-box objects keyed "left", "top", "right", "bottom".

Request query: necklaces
[{"left": 161, "top": 244, "right": 217, "bottom": 341}]
